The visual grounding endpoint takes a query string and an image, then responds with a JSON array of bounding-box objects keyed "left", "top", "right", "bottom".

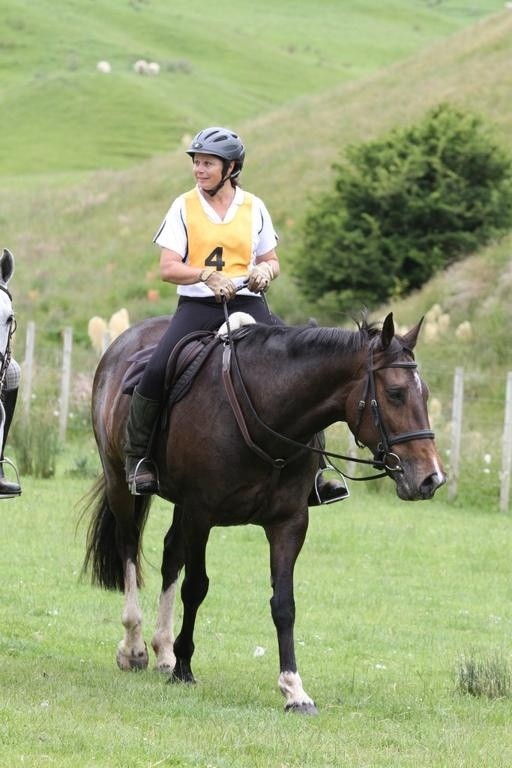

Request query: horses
[
  {"left": 0, "top": 247, "right": 15, "bottom": 464},
  {"left": 76, "top": 312, "right": 448, "bottom": 715}
]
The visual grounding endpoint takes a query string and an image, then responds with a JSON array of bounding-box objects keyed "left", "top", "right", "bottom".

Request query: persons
[
  {"left": 0, "top": 356, "right": 23, "bottom": 495},
  {"left": 121, "top": 125, "right": 350, "bottom": 506}
]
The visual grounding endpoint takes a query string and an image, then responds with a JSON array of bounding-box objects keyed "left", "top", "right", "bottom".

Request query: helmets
[{"left": 187, "top": 127, "right": 243, "bottom": 181}]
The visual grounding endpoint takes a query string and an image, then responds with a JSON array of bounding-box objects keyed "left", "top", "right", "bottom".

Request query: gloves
[
  {"left": 244, "top": 262, "right": 274, "bottom": 294},
  {"left": 199, "top": 270, "right": 237, "bottom": 303}
]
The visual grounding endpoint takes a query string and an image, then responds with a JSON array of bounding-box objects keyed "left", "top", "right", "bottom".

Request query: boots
[
  {"left": 122, "top": 385, "right": 161, "bottom": 495},
  {"left": 308, "top": 473, "right": 345, "bottom": 506},
  {"left": 1, "top": 386, "right": 24, "bottom": 497}
]
